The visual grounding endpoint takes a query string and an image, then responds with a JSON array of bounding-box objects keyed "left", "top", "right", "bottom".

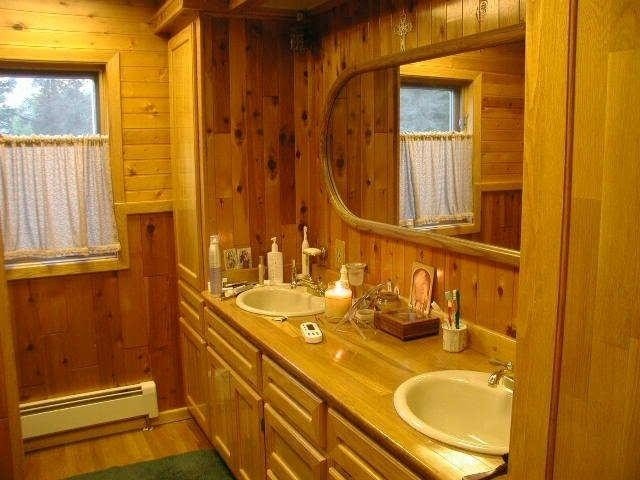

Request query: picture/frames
[{"left": 407, "top": 262, "right": 434, "bottom": 316}]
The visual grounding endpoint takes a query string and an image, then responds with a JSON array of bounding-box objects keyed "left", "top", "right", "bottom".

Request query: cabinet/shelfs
[
  {"left": 202, "top": 304, "right": 427, "bottom": 480},
  {"left": 165, "top": 17, "right": 310, "bottom": 448}
]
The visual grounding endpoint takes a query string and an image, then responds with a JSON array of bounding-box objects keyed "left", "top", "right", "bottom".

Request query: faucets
[
  {"left": 488, "top": 361, "right": 513, "bottom": 387},
  {"left": 288, "top": 280, "right": 311, "bottom": 289}
]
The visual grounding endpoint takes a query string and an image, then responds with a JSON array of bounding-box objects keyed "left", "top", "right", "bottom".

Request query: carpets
[{"left": 71, "top": 446, "right": 236, "bottom": 480}]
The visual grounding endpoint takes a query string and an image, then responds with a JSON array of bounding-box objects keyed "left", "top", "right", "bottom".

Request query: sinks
[
  {"left": 236, "top": 284, "right": 326, "bottom": 316},
  {"left": 394, "top": 370, "right": 513, "bottom": 455}
]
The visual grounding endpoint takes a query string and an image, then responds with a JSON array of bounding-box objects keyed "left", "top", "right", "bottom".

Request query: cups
[
  {"left": 346, "top": 262, "right": 367, "bottom": 286},
  {"left": 324, "top": 286, "right": 352, "bottom": 323},
  {"left": 439, "top": 321, "right": 469, "bottom": 353}
]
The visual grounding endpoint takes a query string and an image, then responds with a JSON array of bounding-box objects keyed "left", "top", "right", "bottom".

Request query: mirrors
[{"left": 317, "top": 21, "right": 523, "bottom": 269}]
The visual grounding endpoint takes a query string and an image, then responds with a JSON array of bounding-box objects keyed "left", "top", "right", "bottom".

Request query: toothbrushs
[
  {"left": 431, "top": 289, "right": 459, "bottom": 330},
  {"left": 302, "top": 226, "right": 309, "bottom": 274}
]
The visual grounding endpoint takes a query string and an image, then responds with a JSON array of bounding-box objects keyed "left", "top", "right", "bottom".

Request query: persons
[
  {"left": 225, "top": 250, "right": 235, "bottom": 269},
  {"left": 240, "top": 250, "right": 249, "bottom": 269},
  {"left": 411, "top": 268, "right": 431, "bottom": 312}
]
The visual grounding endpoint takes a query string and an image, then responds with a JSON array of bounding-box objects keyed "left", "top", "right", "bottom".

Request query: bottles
[
  {"left": 374, "top": 291, "right": 400, "bottom": 311},
  {"left": 207, "top": 235, "right": 222, "bottom": 297},
  {"left": 257, "top": 254, "right": 266, "bottom": 285},
  {"left": 338, "top": 264, "right": 349, "bottom": 290}
]
